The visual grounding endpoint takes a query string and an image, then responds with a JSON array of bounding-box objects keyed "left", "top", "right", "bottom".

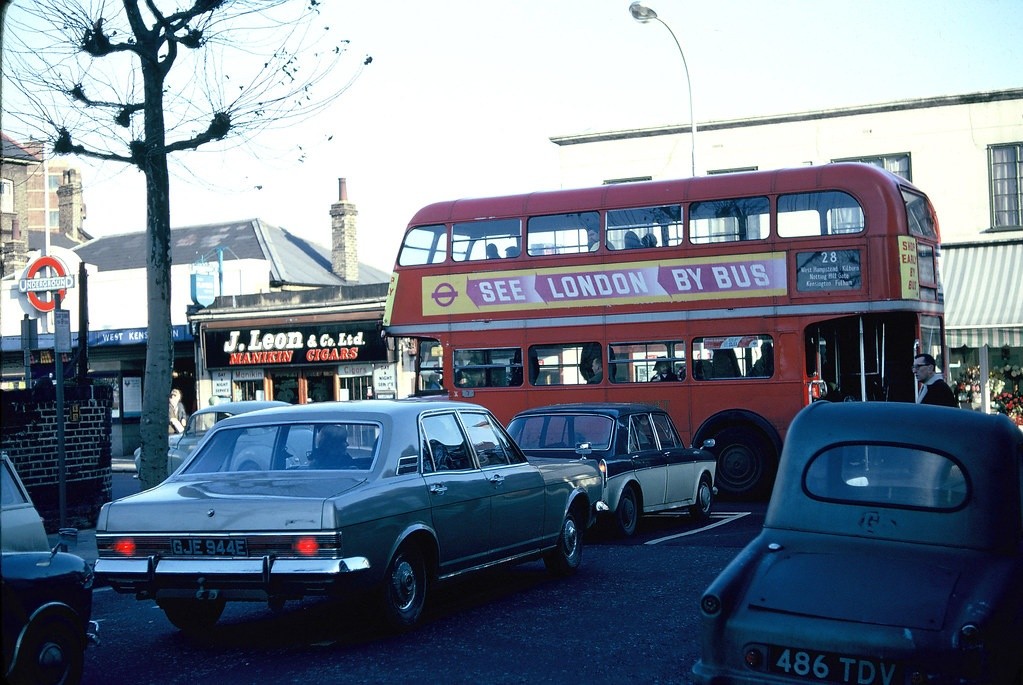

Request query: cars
[
  {"left": 686, "top": 398, "right": 1023, "bottom": 684},
  {"left": 0, "top": 548, "right": 101, "bottom": 684},
  {"left": 491, "top": 400, "right": 720, "bottom": 540},
  {"left": 133, "top": 399, "right": 328, "bottom": 485},
  {"left": 92, "top": 398, "right": 605, "bottom": 630},
  {"left": 0, "top": 450, "right": 53, "bottom": 558}
]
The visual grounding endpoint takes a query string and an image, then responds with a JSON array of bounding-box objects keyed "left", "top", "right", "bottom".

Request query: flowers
[{"left": 953, "top": 364, "right": 1023, "bottom": 426}]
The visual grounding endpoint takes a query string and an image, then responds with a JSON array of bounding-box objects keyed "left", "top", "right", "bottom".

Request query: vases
[{"left": 959, "top": 401, "right": 981, "bottom": 410}]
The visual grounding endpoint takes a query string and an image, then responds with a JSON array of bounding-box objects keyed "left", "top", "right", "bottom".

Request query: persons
[
  {"left": 505, "top": 246, "right": 520, "bottom": 257},
  {"left": 750, "top": 342, "right": 774, "bottom": 376},
  {"left": 510, "top": 347, "right": 539, "bottom": 384},
  {"left": 588, "top": 357, "right": 615, "bottom": 382},
  {"left": 624, "top": 231, "right": 642, "bottom": 247},
  {"left": 486, "top": 243, "right": 501, "bottom": 258},
  {"left": 585, "top": 224, "right": 615, "bottom": 251},
  {"left": 912, "top": 353, "right": 958, "bottom": 407},
  {"left": 712, "top": 348, "right": 742, "bottom": 377},
  {"left": 580, "top": 343, "right": 617, "bottom": 380},
  {"left": 651, "top": 356, "right": 677, "bottom": 381},
  {"left": 168, "top": 389, "right": 187, "bottom": 434},
  {"left": 299, "top": 426, "right": 357, "bottom": 469},
  {"left": 642, "top": 233, "right": 657, "bottom": 246},
  {"left": 427, "top": 373, "right": 440, "bottom": 390}
]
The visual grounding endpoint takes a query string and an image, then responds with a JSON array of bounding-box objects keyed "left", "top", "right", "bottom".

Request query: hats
[{"left": 653, "top": 356, "right": 672, "bottom": 371}]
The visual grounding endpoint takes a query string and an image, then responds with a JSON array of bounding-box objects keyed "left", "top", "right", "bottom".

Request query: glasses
[{"left": 911, "top": 363, "right": 928, "bottom": 370}]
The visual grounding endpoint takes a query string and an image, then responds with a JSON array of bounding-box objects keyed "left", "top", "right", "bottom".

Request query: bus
[{"left": 377, "top": 161, "right": 953, "bottom": 500}]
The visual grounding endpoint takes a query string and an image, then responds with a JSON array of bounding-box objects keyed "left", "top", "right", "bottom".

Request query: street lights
[{"left": 628, "top": 1, "right": 696, "bottom": 181}]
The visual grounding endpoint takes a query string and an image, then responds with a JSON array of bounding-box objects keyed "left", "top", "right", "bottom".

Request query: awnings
[{"left": 920, "top": 242, "right": 1023, "bottom": 348}]
